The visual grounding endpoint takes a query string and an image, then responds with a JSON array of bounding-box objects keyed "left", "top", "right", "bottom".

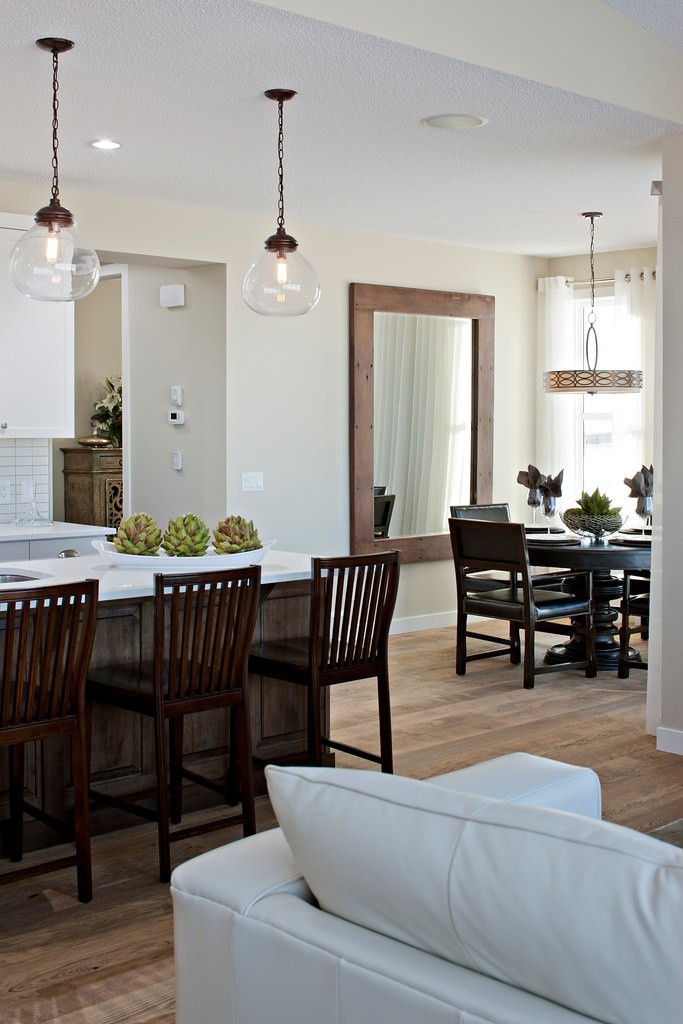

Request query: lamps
[
  {"left": 544, "top": 212, "right": 643, "bottom": 396},
  {"left": 242, "top": 88, "right": 321, "bottom": 319},
  {"left": 8, "top": 38, "right": 102, "bottom": 300}
]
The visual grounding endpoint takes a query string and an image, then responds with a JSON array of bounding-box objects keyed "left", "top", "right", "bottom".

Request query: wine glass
[
  {"left": 635, "top": 497, "right": 652, "bottom": 540},
  {"left": 527, "top": 488, "right": 557, "bottom": 538}
]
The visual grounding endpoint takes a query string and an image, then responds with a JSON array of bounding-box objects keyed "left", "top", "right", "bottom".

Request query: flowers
[{"left": 95, "top": 373, "right": 122, "bottom": 448}]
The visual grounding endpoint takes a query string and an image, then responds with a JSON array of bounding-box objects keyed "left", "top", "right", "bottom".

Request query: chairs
[
  {"left": 618, "top": 569, "right": 651, "bottom": 680},
  {"left": 0, "top": 578, "right": 91, "bottom": 905},
  {"left": 452, "top": 503, "right": 571, "bottom": 656},
  {"left": 81, "top": 564, "right": 257, "bottom": 885},
  {"left": 231, "top": 546, "right": 401, "bottom": 804},
  {"left": 448, "top": 516, "right": 598, "bottom": 690}
]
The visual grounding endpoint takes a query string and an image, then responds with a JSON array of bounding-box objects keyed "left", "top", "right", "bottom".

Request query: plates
[
  {"left": 90, "top": 538, "right": 276, "bottom": 570},
  {"left": 617, "top": 525, "right": 651, "bottom": 542},
  {"left": 525, "top": 523, "right": 579, "bottom": 541}
]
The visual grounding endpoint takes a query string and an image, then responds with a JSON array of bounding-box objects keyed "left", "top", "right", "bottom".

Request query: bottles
[{"left": 10, "top": 481, "right": 54, "bottom": 527}]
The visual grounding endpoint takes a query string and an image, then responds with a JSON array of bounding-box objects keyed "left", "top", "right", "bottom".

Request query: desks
[{"left": 518, "top": 525, "right": 651, "bottom": 670}]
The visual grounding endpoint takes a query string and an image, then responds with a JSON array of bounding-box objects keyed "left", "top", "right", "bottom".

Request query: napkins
[
  {"left": 539, "top": 468, "right": 563, "bottom": 498},
  {"left": 517, "top": 464, "right": 547, "bottom": 490},
  {"left": 623, "top": 464, "right": 654, "bottom": 498}
]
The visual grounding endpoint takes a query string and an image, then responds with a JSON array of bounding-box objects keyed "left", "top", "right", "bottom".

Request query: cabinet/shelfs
[{"left": 61, "top": 450, "right": 122, "bottom": 541}]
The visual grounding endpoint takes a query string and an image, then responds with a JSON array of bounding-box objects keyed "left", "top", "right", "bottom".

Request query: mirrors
[{"left": 350, "top": 281, "right": 496, "bottom": 566}]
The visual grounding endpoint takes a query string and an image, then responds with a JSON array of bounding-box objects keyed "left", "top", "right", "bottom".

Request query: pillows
[{"left": 263, "top": 763, "right": 682, "bottom": 1022}]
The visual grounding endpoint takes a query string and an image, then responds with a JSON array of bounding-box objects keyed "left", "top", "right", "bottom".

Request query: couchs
[{"left": 167, "top": 753, "right": 682, "bottom": 1024}]
